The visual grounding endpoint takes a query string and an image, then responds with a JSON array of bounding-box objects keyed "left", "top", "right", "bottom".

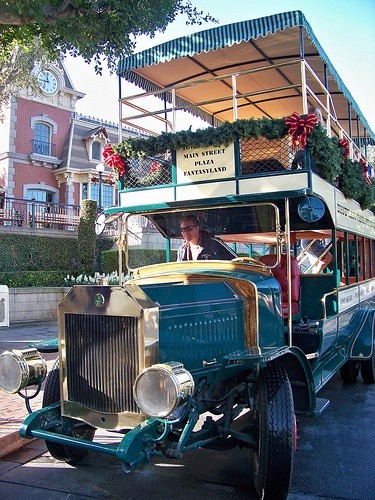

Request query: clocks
[{"left": 35, "top": 69, "right": 58, "bottom": 96}]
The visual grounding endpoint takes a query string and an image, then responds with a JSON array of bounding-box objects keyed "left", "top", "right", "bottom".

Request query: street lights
[{"left": 95, "top": 163, "right": 105, "bottom": 208}]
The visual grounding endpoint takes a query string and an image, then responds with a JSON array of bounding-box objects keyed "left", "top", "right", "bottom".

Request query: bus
[{"left": 0, "top": 10, "right": 375, "bottom": 500}]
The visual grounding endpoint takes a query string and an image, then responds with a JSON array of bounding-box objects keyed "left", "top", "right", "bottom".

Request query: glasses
[{"left": 180, "top": 225, "right": 198, "bottom": 232}]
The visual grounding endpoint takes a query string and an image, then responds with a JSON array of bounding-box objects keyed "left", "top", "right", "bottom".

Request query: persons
[
  {"left": 298, "top": 238, "right": 333, "bottom": 274},
  {"left": 42, "top": 202, "right": 53, "bottom": 229},
  {"left": 177, "top": 215, "right": 239, "bottom": 261}
]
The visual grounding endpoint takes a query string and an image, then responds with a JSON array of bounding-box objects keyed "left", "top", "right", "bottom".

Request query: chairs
[{"left": 256, "top": 254, "right": 304, "bottom": 325}]
